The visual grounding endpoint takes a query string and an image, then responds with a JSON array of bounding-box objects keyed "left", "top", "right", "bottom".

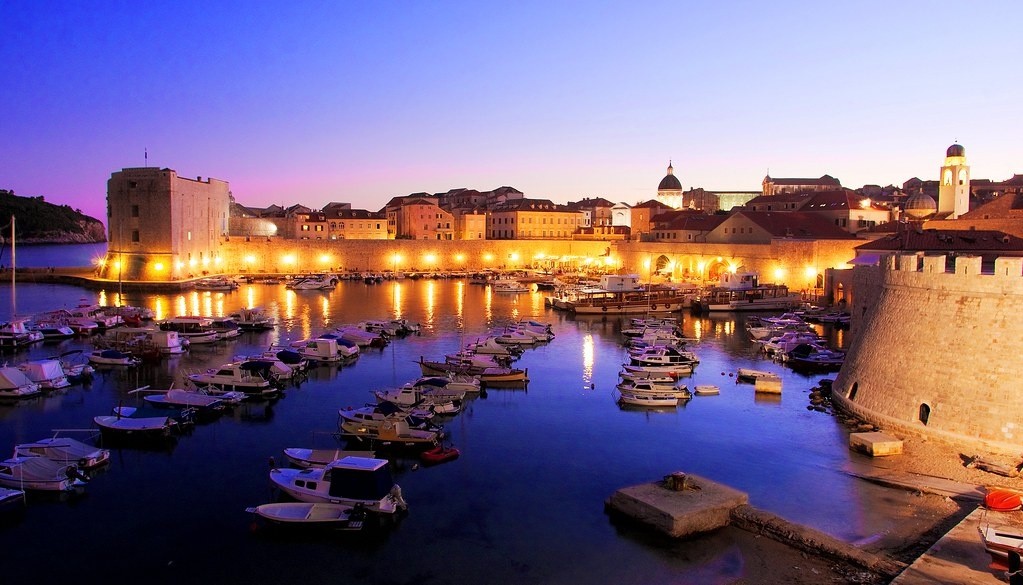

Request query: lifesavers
[
  {"left": 786, "top": 302, "right": 792, "bottom": 308},
  {"left": 729, "top": 304, "right": 735, "bottom": 309},
  {"left": 602, "top": 307, "right": 608, "bottom": 311},
  {"left": 665, "top": 303, "right": 670, "bottom": 309},
  {"left": 599, "top": 285, "right": 603, "bottom": 290},
  {"left": 776, "top": 302, "right": 781, "bottom": 307},
  {"left": 651, "top": 304, "right": 657, "bottom": 310},
  {"left": 617, "top": 306, "right": 621, "bottom": 309},
  {"left": 78, "top": 458, "right": 87, "bottom": 466}
]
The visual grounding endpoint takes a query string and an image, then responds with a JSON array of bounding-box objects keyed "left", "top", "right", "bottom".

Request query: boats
[
  {"left": 245, "top": 502, "right": 370, "bottom": 541},
  {"left": 536, "top": 275, "right": 688, "bottom": 406},
  {"left": 282, "top": 320, "right": 556, "bottom": 468},
  {"left": 0, "top": 308, "right": 278, "bottom": 397},
  {"left": 269, "top": 456, "right": 408, "bottom": 514},
  {"left": 0, "top": 304, "right": 154, "bottom": 346},
  {"left": 682, "top": 274, "right": 851, "bottom": 366},
  {"left": 192, "top": 272, "right": 530, "bottom": 292},
  {"left": 977, "top": 508, "right": 1023, "bottom": 585},
  {"left": 0, "top": 319, "right": 420, "bottom": 513}
]
